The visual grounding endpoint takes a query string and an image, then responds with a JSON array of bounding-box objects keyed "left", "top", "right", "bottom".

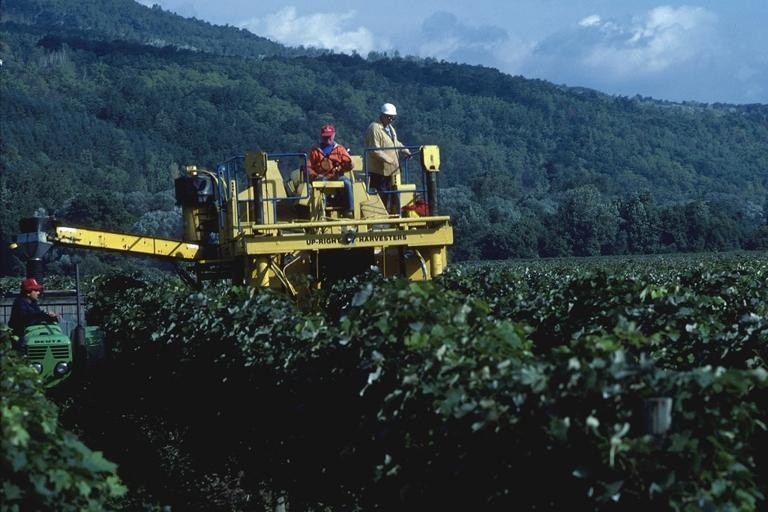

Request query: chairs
[
  {"left": 236, "top": 160, "right": 294, "bottom": 233},
  {"left": 340, "top": 154, "right": 390, "bottom": 222}
]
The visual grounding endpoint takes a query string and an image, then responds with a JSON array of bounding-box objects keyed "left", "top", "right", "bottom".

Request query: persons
[
  {"left": 7, "top": 277, "right": 61, "bottom": 353},
  {"left": 361, "top": 102, "right": 413, "bottom": 232},
  {"left": 305, "top": 123, "right": 354, "bottom": 218}
]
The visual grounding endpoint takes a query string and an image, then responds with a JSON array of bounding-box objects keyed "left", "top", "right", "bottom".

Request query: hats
[
  {"left": 381, "top": 103, "right": 398, "bottom": 116},
  {"left": 321, "top": 125, "right": 335, "bottom": 137},
  {"left": 20, "top": 278, "right": 43, "bottom": 290}
]
